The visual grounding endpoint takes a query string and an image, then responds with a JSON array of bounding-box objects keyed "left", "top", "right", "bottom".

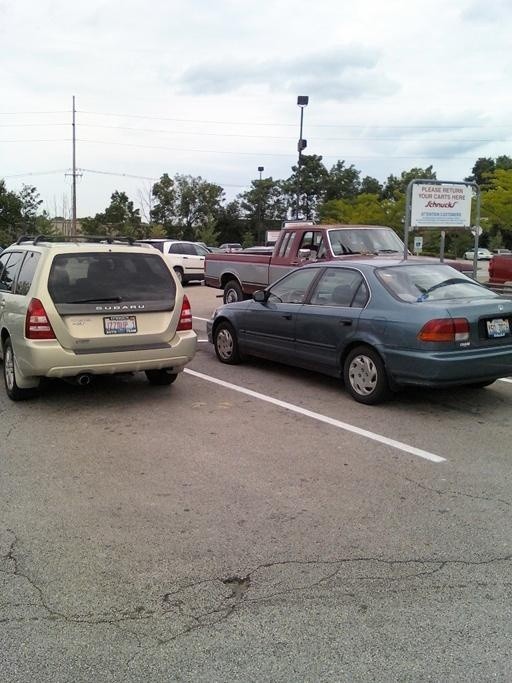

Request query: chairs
[{"left": 322, "top": 283, "right": 417, "bottom": 309}]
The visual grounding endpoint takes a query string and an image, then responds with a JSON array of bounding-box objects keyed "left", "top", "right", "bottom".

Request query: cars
[
  {"left": 207, "top": 261, "right": 512, "bottom": 405},
  {"left": 463, "top": 248, "right": 512, "bottom": 261}
]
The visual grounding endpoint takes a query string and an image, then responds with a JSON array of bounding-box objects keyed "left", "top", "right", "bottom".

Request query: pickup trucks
[{"left": 204, "top": 225, "right": 412, "bottom": 304}]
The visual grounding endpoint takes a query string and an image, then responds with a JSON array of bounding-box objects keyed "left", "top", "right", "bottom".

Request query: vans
[
  {"left": 135, "top": 238, "right": 243, "bottom": 285},
  {"left": 0, "top": 234, "right": 198, "bottom": 401}
]
